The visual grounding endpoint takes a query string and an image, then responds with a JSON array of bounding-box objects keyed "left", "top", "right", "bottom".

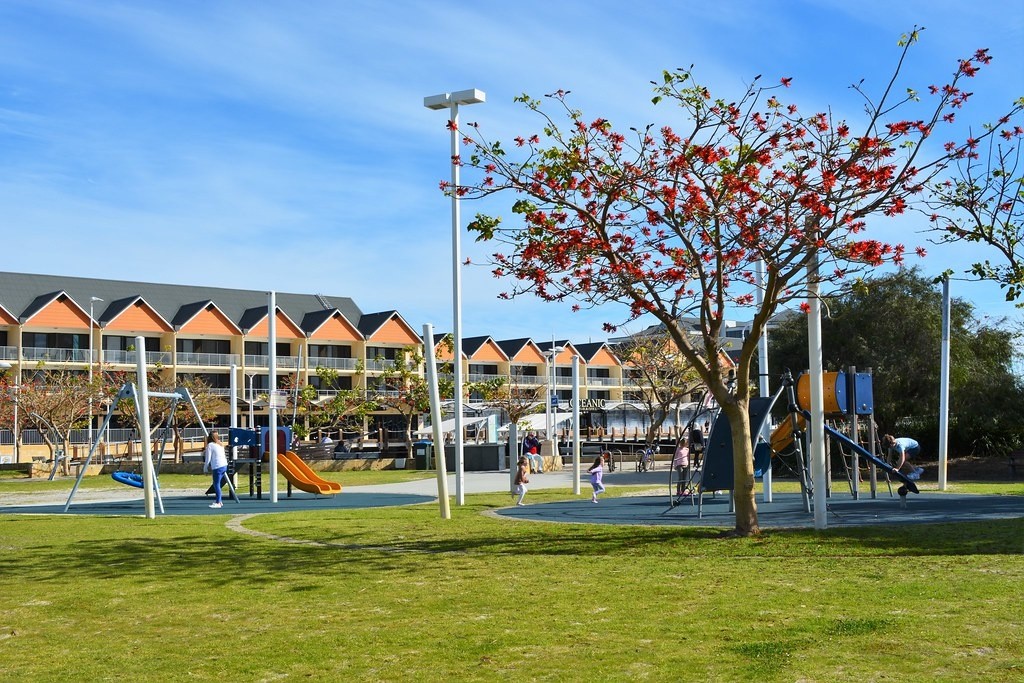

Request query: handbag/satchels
[{"left": 694, "top": 443, "right": 702, "bottom": 451}]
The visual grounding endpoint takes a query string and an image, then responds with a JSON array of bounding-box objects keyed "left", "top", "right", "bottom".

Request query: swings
[{"left": 111, "top": 397, "right": 175, "bottom": 490}]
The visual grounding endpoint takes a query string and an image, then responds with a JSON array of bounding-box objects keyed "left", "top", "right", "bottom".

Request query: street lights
[
  {"left": 421, "top": 88, "right": 487, "bottom": 506},
  {"left": 87, "top": 296, "right": 105, "bottom": 457}
]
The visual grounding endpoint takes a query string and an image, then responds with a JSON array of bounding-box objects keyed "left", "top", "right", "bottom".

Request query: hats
[
  {"left": 325, "top": 432, "right": 330, "bottom": 435},
  {"left": 690, "top": 422, "right": 701, "bottom": 431}
]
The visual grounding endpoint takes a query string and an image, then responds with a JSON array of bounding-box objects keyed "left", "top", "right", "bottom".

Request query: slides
[
  {"left": 265, "top": 451, "right": 342, "bottom": 501},
  {"left": 802, "top": 408, "right": 921, "bottom": 495},
  {"left": 770, "top": 411, "right": 807, "bottom": 458}
]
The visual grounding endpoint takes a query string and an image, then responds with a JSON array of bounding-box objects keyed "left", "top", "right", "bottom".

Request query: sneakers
[
  {"left": 916, "top": 468, "right": 924, "bottom": 475},
  {"left": 906, "top": 472, "right": 921, "bottom": 479},
  {"left": 212, "top": 502, "right": 223, "bottom": 506},
  {"left": 209, "top": 505, "right": 222, "bottom": 509}
]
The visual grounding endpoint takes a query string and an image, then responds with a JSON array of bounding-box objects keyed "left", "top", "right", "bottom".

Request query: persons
[
  {"left": 203, "top": 431, "right": 228, "bottom": 508},
  {"left": 882, "top": 434, "right": 925, "bottom": 480},
  {"left": 321, "top": 432, "right": 333, "bottom": 443},
  {"left": 334, "top": 441, "right": 348, "bottom": 460},
  {"left": 523, "top": 431, "right": 545, "bottom": 474},
  {"left": 511, "top": 455, "right": 530, "bottom": 506},
  {"left": 673, "top": 438, "right": 690, "bottom": 495},
  {"left": 689, "top": 422, "right": 704, "bottom": 467},
  {"left": 705, "top": 421, "right": 709, "bottom": 433},
  {"left": 587, "top": 456, "right": 605, "bottom": 504},
  {"left": 644, "top": 444, "right": 652, "bottom": 458}
]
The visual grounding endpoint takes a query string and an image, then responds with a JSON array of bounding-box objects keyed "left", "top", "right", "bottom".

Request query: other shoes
[
  {"left": 677, "top": 492, "right": 683, "bottom": 495},
  {"left": 714, "top": 491, "right": 718, "bottom": 494},
  {"left": 537, "top": 470, "right": 544, "bottom": 474},
  {"left": 718, "top": 490, "right": 723, "bottom": 495},
  {"left": 592, "top": 492, "right": 596, "bottom": 499},
  {"left": 533, "top": 470, "right": 538, "bottom": 474},
  {"left": 591, "top": 501, "right": 598, "bottom": 504},
  {"left": 682, "top": 492, "right": 688, "bottom": 496}
]
